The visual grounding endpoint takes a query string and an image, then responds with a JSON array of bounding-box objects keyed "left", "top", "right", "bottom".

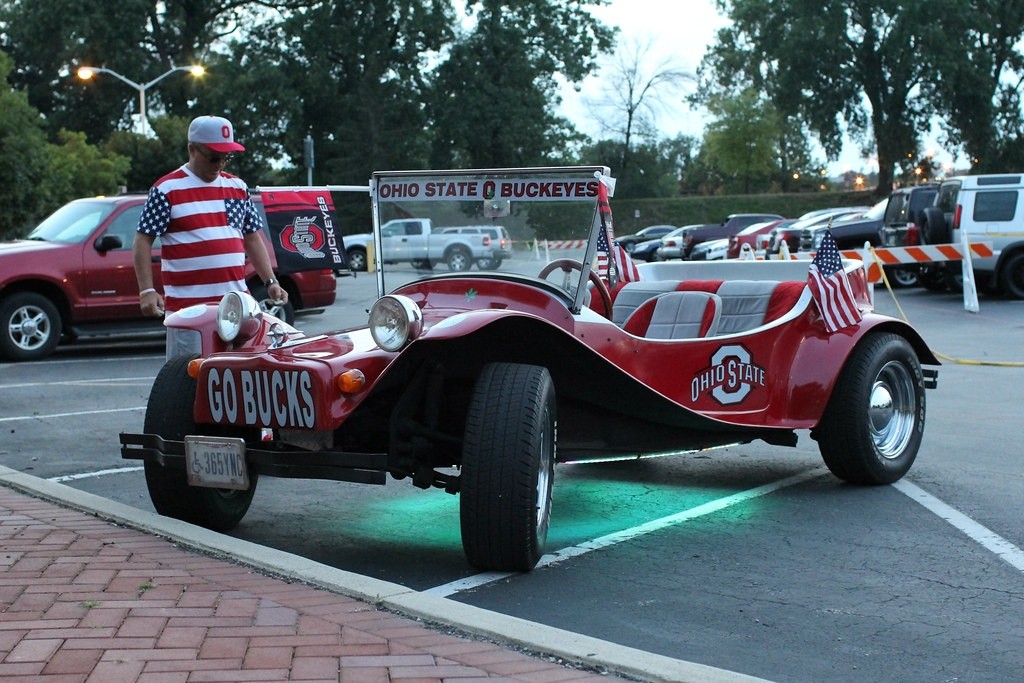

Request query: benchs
[{"left": 585, "top": 277, "right": 807, "bottom": 337}]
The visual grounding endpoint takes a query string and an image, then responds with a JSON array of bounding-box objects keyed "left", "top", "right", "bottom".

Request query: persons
[{"left": 129, "top": 116, "right": 290, "bottom": 365}]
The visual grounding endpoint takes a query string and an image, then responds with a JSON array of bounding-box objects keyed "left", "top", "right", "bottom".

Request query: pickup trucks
[{"left": 342, "top": 218, "right": 494, "bottom": 273}]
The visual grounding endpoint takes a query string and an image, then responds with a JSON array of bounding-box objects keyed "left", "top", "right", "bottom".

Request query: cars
[
  {"left": 630, "top": 207, "right": 869, "bottom": 262},
  {"left": 797, "top": 196, "right": 890, "bottom": 253},
  {"left": 615, "top": 224, "right": 678, "bottom": 254},
  {"left": 119, "top": 162, "right": 943, "bottom": 575}
]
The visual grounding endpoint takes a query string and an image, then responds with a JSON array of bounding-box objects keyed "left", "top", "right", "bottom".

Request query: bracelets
[
  {"left": 264, "top": 277, "right": 280, "bottom": 288},
  {"left": 139, "top": 288, "right": 156, "bottom": 296}
]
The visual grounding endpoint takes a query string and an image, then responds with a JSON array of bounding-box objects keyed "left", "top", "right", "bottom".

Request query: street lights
[{"left": 77, "top": 62, "right": 207, "bottom": 195}]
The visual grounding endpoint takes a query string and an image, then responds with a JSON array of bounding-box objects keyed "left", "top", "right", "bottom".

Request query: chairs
[{"left": 619, "top": 290, "right": 722, "bottom": 338}]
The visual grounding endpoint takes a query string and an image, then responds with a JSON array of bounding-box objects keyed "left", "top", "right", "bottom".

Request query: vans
[{"left": 410, "top": 226, "right": 513, "bottom": 271}]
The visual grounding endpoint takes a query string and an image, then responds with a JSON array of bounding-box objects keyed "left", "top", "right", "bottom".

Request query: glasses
[{"left": 192, "top": 143, "right": 230, "bottom": 164}]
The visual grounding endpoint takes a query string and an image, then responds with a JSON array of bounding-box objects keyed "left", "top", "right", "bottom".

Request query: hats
[{"left": 188, "top": 114, "right": 245, "bottom": 153}]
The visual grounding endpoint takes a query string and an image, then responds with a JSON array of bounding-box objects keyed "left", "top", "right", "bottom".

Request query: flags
[
  {"left": 807, "top": 230, "right": 863, "bottom": 334},
  {"left": 594, "top": 222, "right": 640, "bottom": 282}
]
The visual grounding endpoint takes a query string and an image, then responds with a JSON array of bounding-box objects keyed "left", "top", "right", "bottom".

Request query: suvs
[
  {"left": 918, "top": 173, "right": 1024, "bottom": 300},
  {"left": 0, "top": 186, "right": 346, "bottom": 363},
  {"left": 879, "top": 181, "right": 941, "bottom": 289}
]
